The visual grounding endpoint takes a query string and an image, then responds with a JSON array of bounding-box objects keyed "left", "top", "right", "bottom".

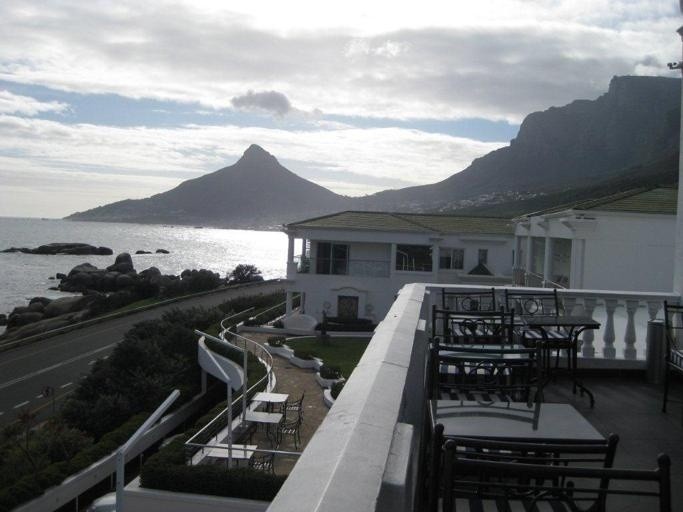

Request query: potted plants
[
  {"left": 290, "top": 347, "right": 322, "bottom": 369},
  {"left": 323, "top": 380, "right": 345, "bottom": 408},
  {"left": 316, "top": 365, "right": 345, "bottom": 388},
  {"left": 264, "top": 336, "right": 289, "bottom": 354}
]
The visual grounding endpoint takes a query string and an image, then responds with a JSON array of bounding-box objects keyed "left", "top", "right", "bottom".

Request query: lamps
[
  {"left": 275, "top": 418, "right": 303, "bottom": 450},
  {"left": 251, "top": 453, "right": 275, "bottom": 474},
  {"left": 283, "top": 393, "right": 304, "bottom": 424}
]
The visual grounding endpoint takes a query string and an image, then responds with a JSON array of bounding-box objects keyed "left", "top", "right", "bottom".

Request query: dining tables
[
  {"left": 316, "top": 365, "right": 345, "bottom": 388},
  {"left": 323, "top": 380, "right": 345, "bottom": 408},
  {"left": 290, "top": 347, "right": 322, "bottom": 369},
  {"left": 264, "top": 336, "right": 289, "bottom": 354}
]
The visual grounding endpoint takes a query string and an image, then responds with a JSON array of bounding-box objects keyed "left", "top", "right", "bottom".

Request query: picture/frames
[
  {"left": 251, "top": 392, "right": 289, "bottom": 412},
  {"left": 207, "top": 443, "right": 258, "bottom": 468},
  {"left": 245, "top": 412, "right": 283, "bottom": 441}
]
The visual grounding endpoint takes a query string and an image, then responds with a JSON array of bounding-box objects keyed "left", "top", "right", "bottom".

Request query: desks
[
  {"left": 251, "top": 392, "right": 289, "bottom": 412},
  {"left": 207, "top": 443, "right": 258, "bottom": 468},
  {"left": 245, "top": 412, "right": 283, "bottom": 441}
]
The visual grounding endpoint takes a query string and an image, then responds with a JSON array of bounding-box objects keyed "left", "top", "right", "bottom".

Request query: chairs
[
  {"left": 275, "top": 418, "right": 303, "bottom": 450},
  {"left": 251, "top": 453, "right": 275, "bottom": 474},
  {"left": 423, "top": 288, "right": 620, "bottom": 512},
  {"left": 662, "top": 300, "right": 682, "bottom": 414},
  {"left": 283, "top": 393, "right": 304, "bottom": 424},
  {"left": 441, "top": 439, "right": 672, "bottom": 508}
]
